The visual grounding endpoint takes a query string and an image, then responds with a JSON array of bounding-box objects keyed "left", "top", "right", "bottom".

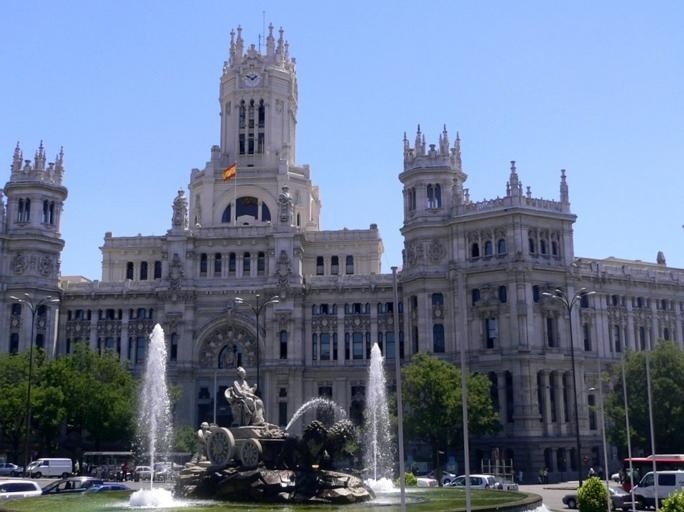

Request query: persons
[
  {"left": 74, "top": 459, "right": 80, "bottom": 476},
  {"left": 197, "top": 421, "right": 214, "bottom": 463},
  {"left": 543, "top": 466, "right": 548, "bottom": 483},
  {"left": 619, "top": 466, "right": 625, "bottom": 484},
  {"left": 538, "top": 465, "right": 549, "bottom": 484},
  {"left": 233, "top": 366, "right": 266, "bottom": 426},
  {"left": 516, "top": 469, "right": 524, "bottom": 484},
  {"left": 120, "top": 461, "right": 129, "bottom": 482},
  {"left": 588, "top": 465, "right": 594, "bottom": 478}
]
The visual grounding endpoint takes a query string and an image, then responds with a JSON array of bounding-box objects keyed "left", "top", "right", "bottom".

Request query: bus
[{"left": 620, "top": 453, "right": 684, "bottom": 492}]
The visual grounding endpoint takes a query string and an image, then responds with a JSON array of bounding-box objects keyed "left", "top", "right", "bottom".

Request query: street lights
[
  {"left": 541, "top": 287, "right": 597, "bottom": 487},
  {"left": 234, "top": 294, "right": 280, "bottom": 396},
  {"left": 9, "top": 292, "right": 61, "bottom": 479}
]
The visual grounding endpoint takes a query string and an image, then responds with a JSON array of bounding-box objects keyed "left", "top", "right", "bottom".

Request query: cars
[
  {"left": 82, "top": 451, "right": 193, "bottom": 482},
  {"left": 0, "top": 462, "right": 23, "bottom": 477},
  {"left": 0, "top": 476, "right": 131, "bottom": 500},
  {"left": 416, "top": 469, "right": 519, "bottom": 492},
  {"left": 562, "top": 486, "right": 640, "bottom": 512}
]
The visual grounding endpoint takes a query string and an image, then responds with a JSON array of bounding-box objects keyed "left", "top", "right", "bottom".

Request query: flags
[{"left": 224, "top": 161, "right": 236, "bottom": 181}]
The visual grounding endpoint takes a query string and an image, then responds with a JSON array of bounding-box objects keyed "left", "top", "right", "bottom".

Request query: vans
[
  {"left": 25, "top": 457, "right": 73, "bottom": 479},
  {"left": 629, "top": 470, "right": 684, "bottom": 510}
]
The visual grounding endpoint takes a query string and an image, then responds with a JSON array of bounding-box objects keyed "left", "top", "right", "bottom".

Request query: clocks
[{"left": 239, "top": 66, "right": 264, "bottom": 88}]
